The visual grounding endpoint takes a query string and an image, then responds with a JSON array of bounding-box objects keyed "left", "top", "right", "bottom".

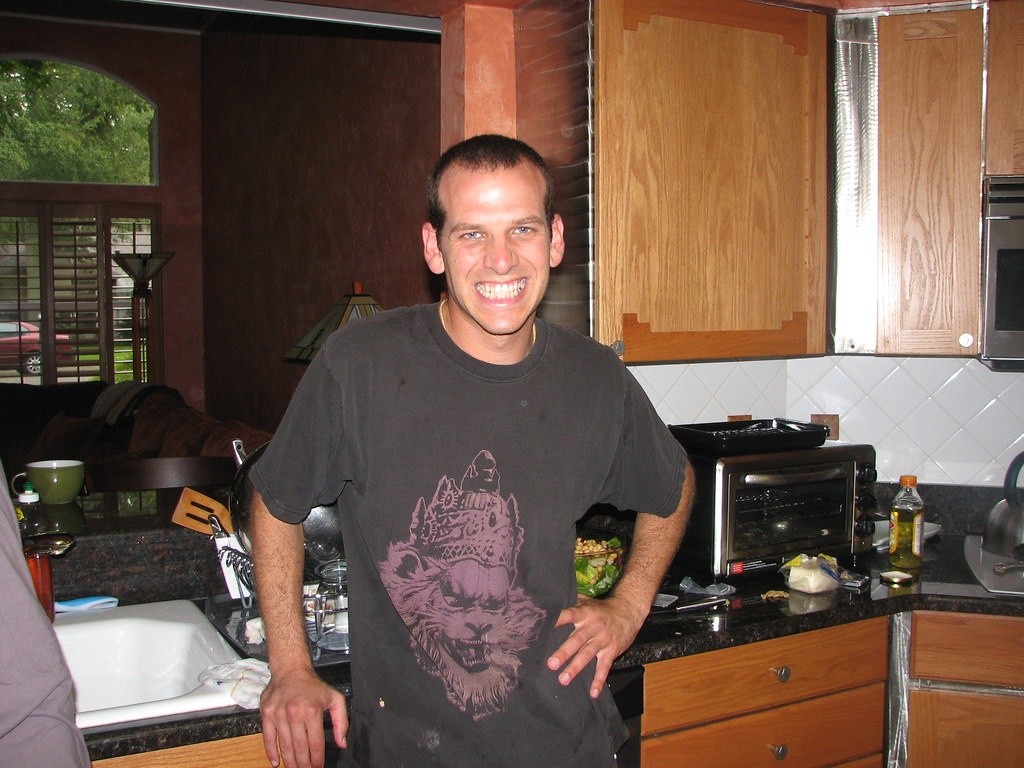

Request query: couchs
[{"left": 0, "top": 381, "right": 275, "bottom": 461}]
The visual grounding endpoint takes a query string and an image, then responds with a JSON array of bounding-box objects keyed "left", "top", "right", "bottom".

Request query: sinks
[{"left": 49, "top": 598, "right": 242, "bottom": 728}]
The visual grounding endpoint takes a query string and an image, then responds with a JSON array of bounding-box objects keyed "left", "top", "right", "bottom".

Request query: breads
[{"left": 788, "top": 561, "right": 838, "bottom": 592}]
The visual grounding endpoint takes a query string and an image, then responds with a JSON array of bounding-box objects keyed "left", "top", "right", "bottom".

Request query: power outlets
[{"left": 811, "top": 413, "right": 840, "bottom": 440}]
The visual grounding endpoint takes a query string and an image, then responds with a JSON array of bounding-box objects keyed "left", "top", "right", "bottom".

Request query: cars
[{"left": 0, "top": 320, "right": 77, "bottom": 377}]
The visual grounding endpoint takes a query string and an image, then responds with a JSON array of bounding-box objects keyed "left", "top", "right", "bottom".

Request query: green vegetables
[{"left": 573, "top": 536, "right": 625, "bottom": 596}]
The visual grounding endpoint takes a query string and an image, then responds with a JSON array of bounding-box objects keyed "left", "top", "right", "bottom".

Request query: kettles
[{"left": 981, "top": 451, "right": 1024, "bottom": 558}]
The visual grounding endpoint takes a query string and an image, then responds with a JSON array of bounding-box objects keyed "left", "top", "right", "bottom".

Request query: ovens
[{"left": 675, "top": 439, "right": 878, "bottom": 578}]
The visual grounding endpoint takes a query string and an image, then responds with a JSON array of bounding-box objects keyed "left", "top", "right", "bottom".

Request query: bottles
[
  {"left": 14, "top": 482, "right": 55, "bottom": 624},
  {"left": 313, "top": 558, "right": 350, "bottom": 651},
  {"left": 890, "top": 475, "right": 924, "bottom": 568}
]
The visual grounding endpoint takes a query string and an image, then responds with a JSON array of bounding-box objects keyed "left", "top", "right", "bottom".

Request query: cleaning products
[{"left": 17, "top": 481, "right": 56, "bottom": 622}]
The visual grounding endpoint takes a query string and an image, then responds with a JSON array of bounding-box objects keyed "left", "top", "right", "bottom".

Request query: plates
[{"left": 873, "top": 520, "right": 942, "bottom": 550}]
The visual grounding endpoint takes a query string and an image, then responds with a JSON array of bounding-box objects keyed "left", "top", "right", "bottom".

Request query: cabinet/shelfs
[
  {"left": 898, "top": 611, "right": 1024, "bottom": 768},
  {"left": 640, "top": 616, "right": 894, "bottom": 768},
  {"left": 830, "top": 1, "right": 985, "bottom": 356},
  {"left": 513, "top": 0, "right": 833, "bottom": 362}
]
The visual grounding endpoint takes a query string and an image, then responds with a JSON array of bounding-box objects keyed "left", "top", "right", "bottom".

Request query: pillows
[{"left": 28, "top": 412, "right": 104, "bottom": 467}]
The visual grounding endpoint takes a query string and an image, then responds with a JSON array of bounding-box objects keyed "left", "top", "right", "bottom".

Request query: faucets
[{"left": 16, "top": 530, "right": 75, "bottom": 559}]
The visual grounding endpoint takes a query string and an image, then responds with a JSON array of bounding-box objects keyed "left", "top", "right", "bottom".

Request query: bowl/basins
[{"left": 574, "top": 525, "right": 627, "bottom": 598}]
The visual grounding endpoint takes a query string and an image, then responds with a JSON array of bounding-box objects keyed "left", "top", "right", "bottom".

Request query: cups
[{"left": 11, "top": 460, "right": 84, "bottom": 504}]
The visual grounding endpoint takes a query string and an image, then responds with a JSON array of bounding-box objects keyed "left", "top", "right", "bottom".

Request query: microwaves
[{"left": 981, "top": 176, "right": 1024, "bottom": 373}]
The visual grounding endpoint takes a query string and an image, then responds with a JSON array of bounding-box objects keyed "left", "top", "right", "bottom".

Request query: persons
[
  {"left": 246, "top": 133, "right": 694, "bottom": 765},
  {"left": 0, "top": 460, "right": 95, "bottom": 768}
]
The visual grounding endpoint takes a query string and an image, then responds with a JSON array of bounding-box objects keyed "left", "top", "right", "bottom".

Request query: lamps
[
  {"left": 111, "top": 252, "right": 176, "bottom": 382},
  {"left": 281, "top": 280, "right": 385, "bottom": 364}
]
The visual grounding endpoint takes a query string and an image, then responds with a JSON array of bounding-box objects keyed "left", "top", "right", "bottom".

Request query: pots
[{"left": 228, "top": 440, "right": 345, "bottom": 584}]
